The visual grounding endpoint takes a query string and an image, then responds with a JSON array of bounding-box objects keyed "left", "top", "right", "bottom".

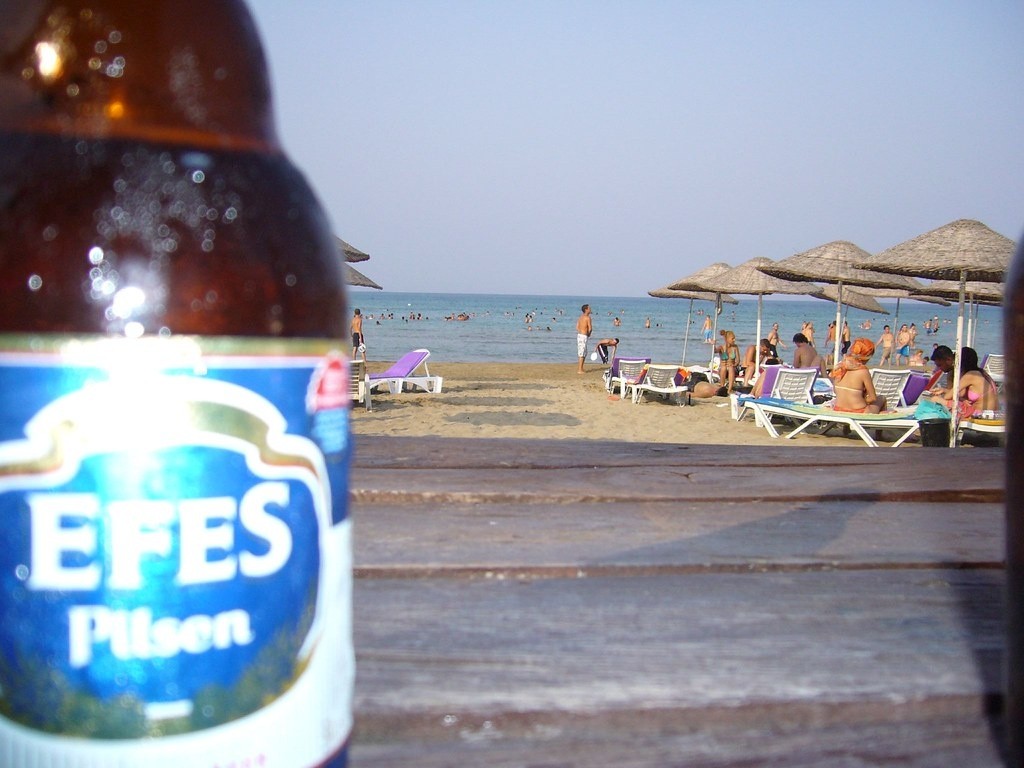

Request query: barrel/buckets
[{"left": 918, "top": 419, "right": 950, "bottom": 448}]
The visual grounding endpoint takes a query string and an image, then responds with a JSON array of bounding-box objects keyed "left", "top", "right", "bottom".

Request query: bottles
[{"left": 0, "top": 1, "right": 354, "bottom": 768}]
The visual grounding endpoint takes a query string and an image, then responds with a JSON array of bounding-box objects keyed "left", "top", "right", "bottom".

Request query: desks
[{"left": 343, "top": 430, "right": 1024, "bottom": 768}]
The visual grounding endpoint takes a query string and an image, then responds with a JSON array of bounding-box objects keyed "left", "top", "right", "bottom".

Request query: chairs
[
  {"left": 367, "top": 348, "right": 443, "bottom": 393},
  {"left": 346, "top": 359, "right": 373, "bottom": 412},
  {"left": 601, "top": 354, "right": 1007, "bottom": 448}
]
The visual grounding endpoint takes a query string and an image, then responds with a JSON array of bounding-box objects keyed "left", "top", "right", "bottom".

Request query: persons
[
  {"left": 350, "top": 309, "right": 366, "bottom": 363},
  {"left": 361, "top": 299, "right": 564, "bottom": 334},
  {"left": 577, "top": 304, "right": 592, "bottom": 374},
  {"left": 742, "top": 339, "right": 772, "bottom": 386},
  {"left": 793, "top": 333, "right": 819, "bottom": 370},
  {"left": 768, "top": 322, "right": 787, "bottom": 362},
  {"left": 595, "top": 307, "right": 737, "bottom": 345},
  {"left": 682, "top": 372, "right": 728, "bottom": 398},
  {"left": 827, "top": 337, "right": 888, "bottom": 441},
  {"left": 710, "top": 329, "right": 740, "bottom": 394},
  {"left": 911, "top": 346, "right": 996, "bottom": 441},
  {"left": 748, "top": 359, "right": 786, "bottom": 398},
  {"left": 593, "top": 338, "right": 619, "bottom": 366},
  {"left": 800, "top": 312, "right": 991, "bottom": 370}
]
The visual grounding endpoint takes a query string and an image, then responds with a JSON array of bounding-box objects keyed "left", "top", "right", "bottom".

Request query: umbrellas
[{"left": 647, "top": 221, "right": 1015, "bottom": 313}]
